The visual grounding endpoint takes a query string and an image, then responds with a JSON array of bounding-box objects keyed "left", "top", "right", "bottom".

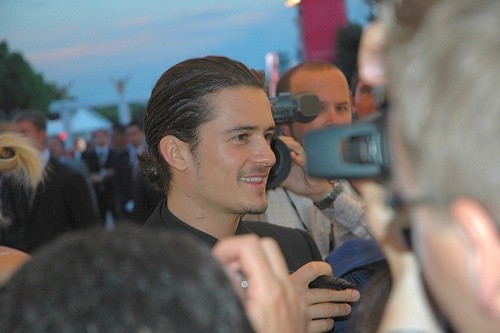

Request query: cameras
[
  {"left": 304, "top": 123, "right": 389, "bottom": 179},
  {"left": 265, "top": 90, "right": 321, "bottom": 189}
]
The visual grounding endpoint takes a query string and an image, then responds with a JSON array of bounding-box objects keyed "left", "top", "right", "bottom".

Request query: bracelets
[{"left": 313, "top": 181, "right": 344, "bottom": 209}]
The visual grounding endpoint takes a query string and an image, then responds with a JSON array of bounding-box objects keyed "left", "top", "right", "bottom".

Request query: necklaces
[{"left": 281, "top": 185, "right": 336, "bottom": 258}]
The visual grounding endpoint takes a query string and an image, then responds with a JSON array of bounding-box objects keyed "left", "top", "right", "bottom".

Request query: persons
[{"left": 0, "top": 0, "right": 499, "bottom": 332}]
